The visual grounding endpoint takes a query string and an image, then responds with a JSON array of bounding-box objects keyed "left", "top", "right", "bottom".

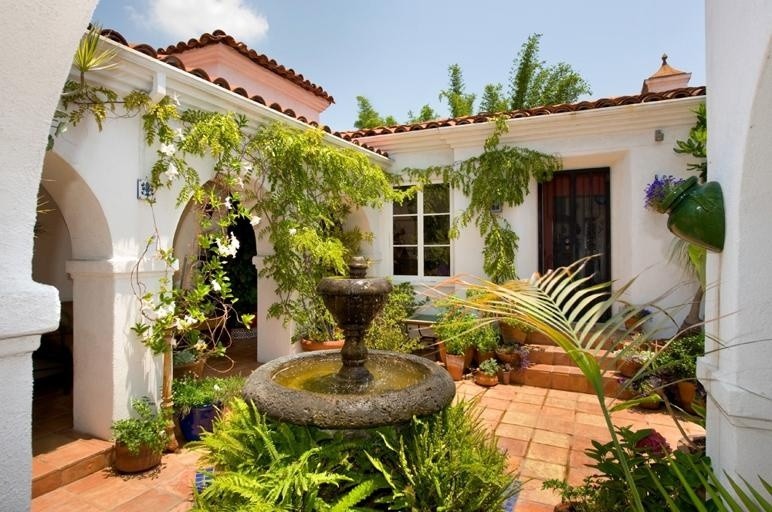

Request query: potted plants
[
  {"left": 541, "top": 473, "right": 599, "bottom": 512},
  {"left": 169, "top": 372, "right": 230, "bottom": 443},
  {"left": 430, "top": 294, "right": 545, "bottom": 388},
  {"left": 236, "top": 119, "right": 418, "bottom": 351},
  {"left": 615, "top": 296, "right": 704, "bottom": 410}
]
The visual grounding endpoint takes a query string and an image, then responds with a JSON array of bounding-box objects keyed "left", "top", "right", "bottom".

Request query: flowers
[{"left": 641, "top": 171, "right": 690, "bottom": 213}]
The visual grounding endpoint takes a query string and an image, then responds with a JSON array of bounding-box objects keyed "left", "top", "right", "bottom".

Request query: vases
[{"left": 657, "top": 175, "right": 726, "bottom": 254}]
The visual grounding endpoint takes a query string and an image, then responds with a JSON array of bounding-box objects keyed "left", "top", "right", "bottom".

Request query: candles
[{"left": 108, "top": 394, "right": 174, "bottom": 475}]
